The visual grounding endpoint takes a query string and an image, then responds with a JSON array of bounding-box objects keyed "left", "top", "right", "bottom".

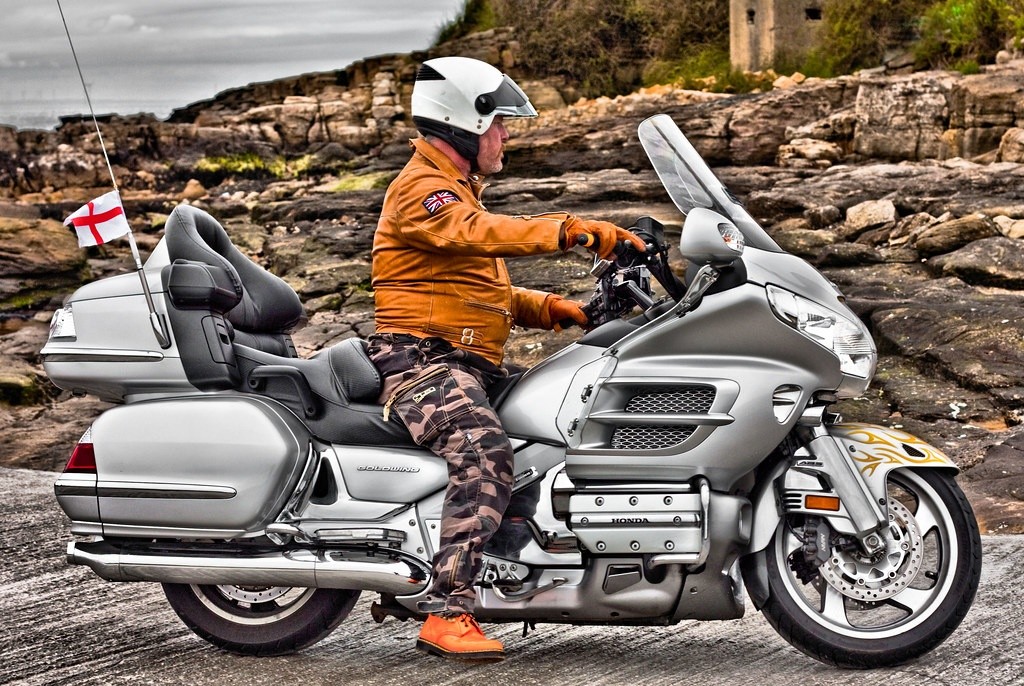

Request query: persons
[{"left": 370, "top": 57, "right": 646, "bottom": 660}]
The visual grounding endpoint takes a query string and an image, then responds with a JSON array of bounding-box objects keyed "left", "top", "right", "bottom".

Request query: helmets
[{"left": 410, "top": 57, "right": 538, "bottom": 176}]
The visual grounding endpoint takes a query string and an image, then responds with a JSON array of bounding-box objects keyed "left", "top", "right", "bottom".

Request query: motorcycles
[{"left": 38, "top": 0, "right": 982, "bottom": 669}]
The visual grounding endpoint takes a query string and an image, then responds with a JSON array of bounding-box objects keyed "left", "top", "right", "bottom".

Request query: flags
[{"left": 62, "top": 189, "right": 129, "bottom": 248}]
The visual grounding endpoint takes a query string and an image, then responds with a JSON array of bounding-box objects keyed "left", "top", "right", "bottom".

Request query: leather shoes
[{"left": 415, "top": 613, "right": 505, "bottom": 663}]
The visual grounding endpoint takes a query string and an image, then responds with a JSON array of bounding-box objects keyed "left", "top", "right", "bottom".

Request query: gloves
[
  {"left": 562, "top": 214, "right": 646, "bottom": 262},
  {"left": 543, "top": 293, "right": 587, "bottom": 329}
]
